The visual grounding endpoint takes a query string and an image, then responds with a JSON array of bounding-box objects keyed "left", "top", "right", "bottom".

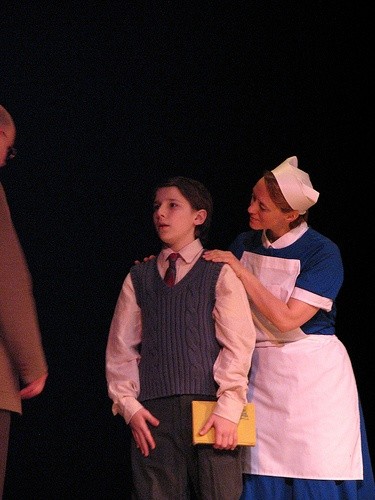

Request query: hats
[{"left": 270, "top": 157, "right": 321, "bottom": 215}]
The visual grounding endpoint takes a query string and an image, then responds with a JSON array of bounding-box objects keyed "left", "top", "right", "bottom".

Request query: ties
[{"left": 162, "top": 253, "right": 180, "bottom": 288}]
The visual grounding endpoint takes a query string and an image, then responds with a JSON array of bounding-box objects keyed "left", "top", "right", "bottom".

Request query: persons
[
  {"left": 0, "top": 104, "right": 48, "bottom": 500},
  {"left": 105, "top": 175, "right": 257, "bottom": 500},
  {"left": 135, "top": 156, "right": 375, "bottom": 500}
]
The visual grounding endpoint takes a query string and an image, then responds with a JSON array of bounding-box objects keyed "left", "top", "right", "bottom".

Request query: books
[{"left": 192, "top": 401, "right": 257, "bottom": 446}]
